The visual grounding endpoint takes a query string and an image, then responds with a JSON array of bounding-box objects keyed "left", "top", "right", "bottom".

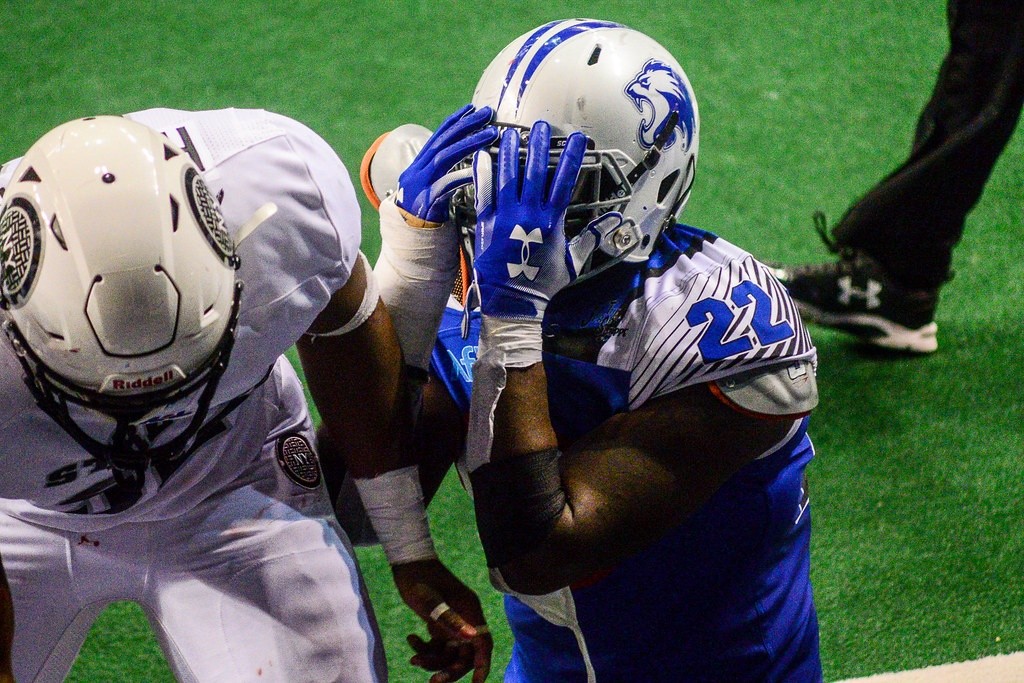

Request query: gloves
[
  {"left": 394, "top": 102, "right": 500, "bottom": 229},
  {"left": 471, "top": 121, "right": 623, "bottom": 323}
]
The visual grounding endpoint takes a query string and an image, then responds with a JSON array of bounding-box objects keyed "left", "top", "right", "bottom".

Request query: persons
[
  {"left": 314, "top": 16, "right": 825, "bottom": 683},
  {"left": 0, "top": 107, "right": 493, "bottom": 683},
  {"left": 762, "top": 0, "right": 1024, "bottom": 353}
]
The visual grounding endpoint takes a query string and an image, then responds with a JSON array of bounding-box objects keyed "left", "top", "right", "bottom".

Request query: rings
[{"left": 430, "top": 601, "right": 450, "bottom": 621}]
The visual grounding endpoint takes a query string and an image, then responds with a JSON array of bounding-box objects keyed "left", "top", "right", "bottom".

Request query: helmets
[
  {"left": 0, "top": 114, "right": 241, "bottom": 471},
  {"left": 468, "top": 19, "right": 701, "bottom": 268}
]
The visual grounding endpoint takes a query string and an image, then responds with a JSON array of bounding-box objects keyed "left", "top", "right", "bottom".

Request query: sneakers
[{"left": 767, "top": 254, "right": 938, "bottom": 350}]
